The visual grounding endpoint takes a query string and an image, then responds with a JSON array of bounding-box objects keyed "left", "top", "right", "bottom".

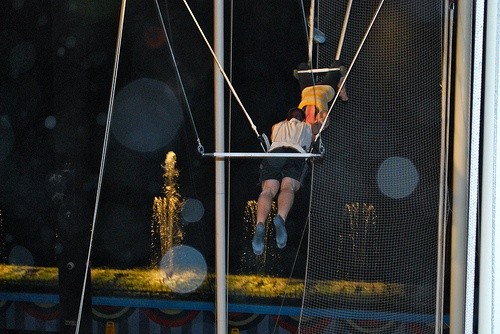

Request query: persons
[
  {"left": 295, "top": 62, "right": 350, "bottom": 140},
  {"left": 253, "top": 107, "right": 324, "bottom": 256}
]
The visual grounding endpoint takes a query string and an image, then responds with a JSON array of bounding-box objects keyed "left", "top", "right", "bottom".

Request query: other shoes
[
  {"left": 274, "top": 214, "right": 288, "bottom": 248},
  {"left": 252, "top": 222, "right": 266, "bottom": 256}
]
